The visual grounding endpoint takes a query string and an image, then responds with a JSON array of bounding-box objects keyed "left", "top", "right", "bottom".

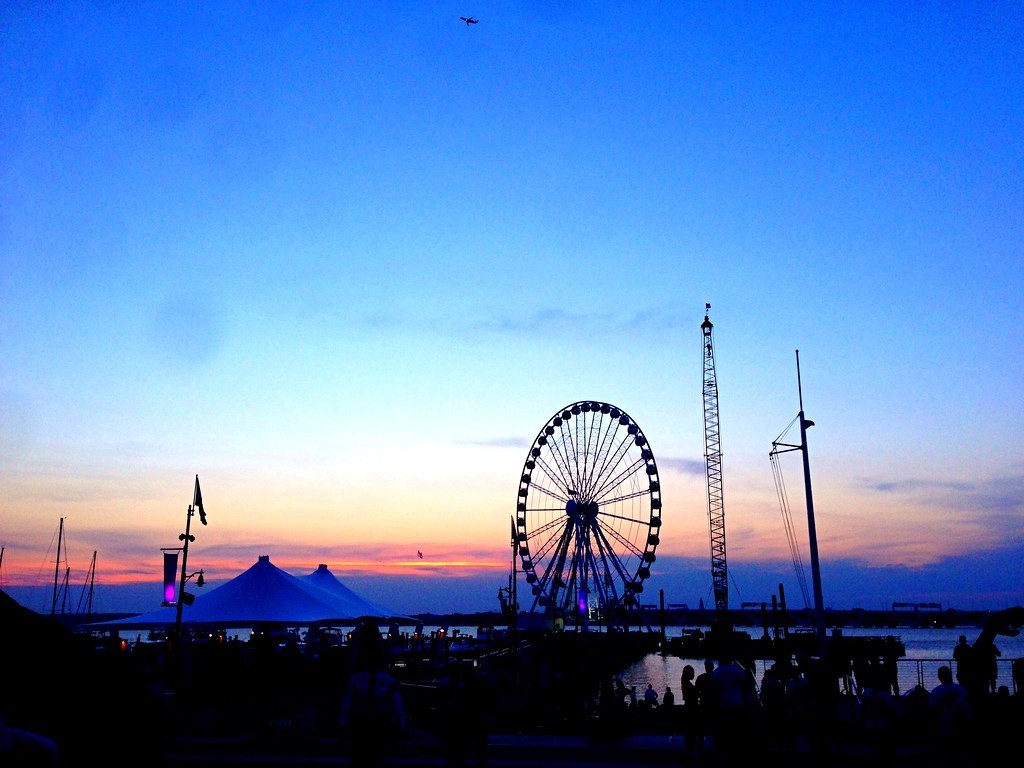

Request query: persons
[{"left": 645, "top": 635, "right": 1024, "bottom": 768}]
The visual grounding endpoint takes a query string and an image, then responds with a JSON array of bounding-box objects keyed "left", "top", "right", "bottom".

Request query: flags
[
  {"left": 706, "top": 303, "right": 712, "bottom": 308},
  {"left": 193, "top": 476, "right": 207, "bottom": 526}
]
